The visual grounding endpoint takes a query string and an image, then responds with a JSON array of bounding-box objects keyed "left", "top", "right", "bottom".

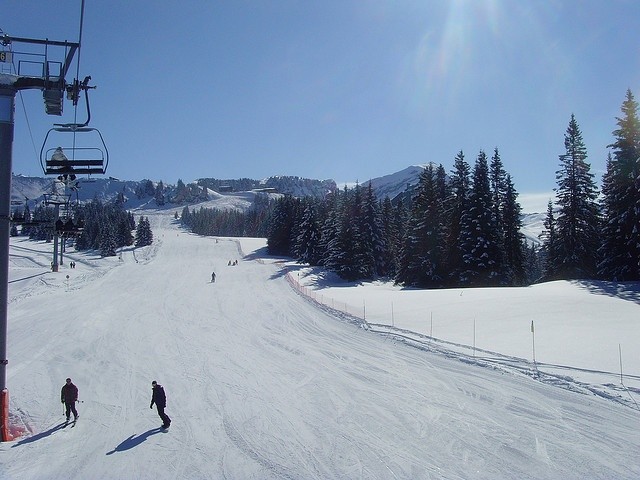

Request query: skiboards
[{"left": 62, "top": 416, "right": 79, "bottom": 428}]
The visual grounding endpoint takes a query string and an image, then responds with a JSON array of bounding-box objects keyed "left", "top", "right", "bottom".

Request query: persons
[
  {"left": 49, "top": 146, "right": 83, "bottom": 192},
  {"left": 61, "top": 378, "right": 79, "bottom": 422},
  {"left": 150, "top": 381, "right": 171, "bottom": 428},
  {"left": 70, "top": 262, "right": 76, "bottom": 269},
  {"left": 212, "top": 271, "right": 216, "bottom": 282}
]
